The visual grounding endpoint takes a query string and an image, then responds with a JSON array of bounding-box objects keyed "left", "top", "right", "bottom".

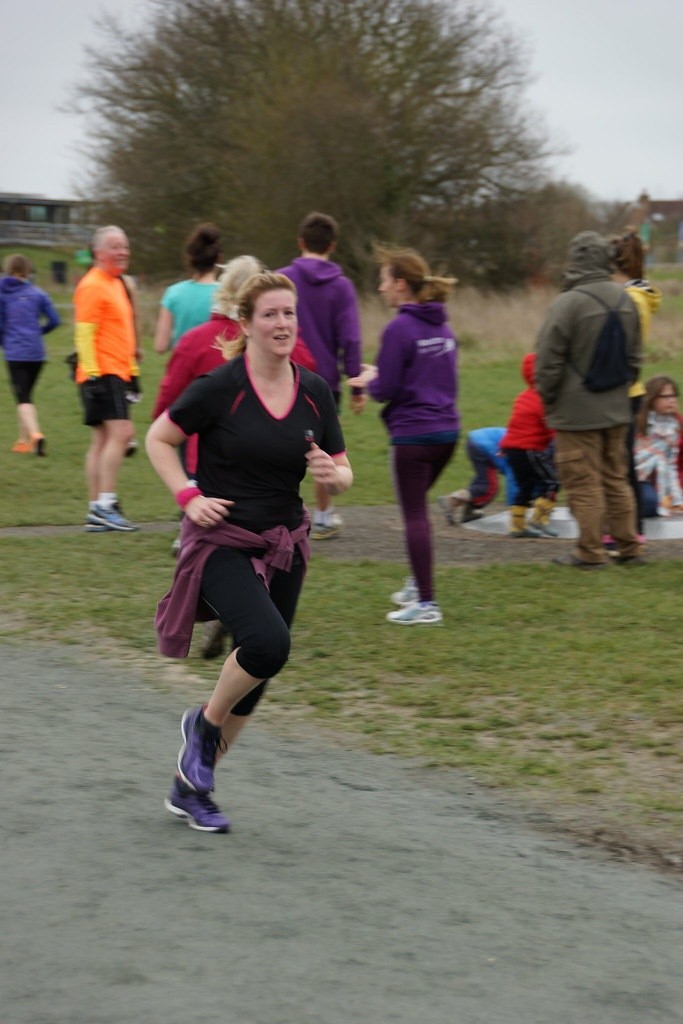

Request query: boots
[
  {"left": 527, "top": 496, "right": 561, "bottom": 537},
  {"left": 508, "top": 505, "right": 541, "bottom": 538}
]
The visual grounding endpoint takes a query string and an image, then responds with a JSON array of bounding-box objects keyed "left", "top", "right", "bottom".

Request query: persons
[
  {"left": 501, "top": 352, "right": 561, "bottom": 538},
  {"left": 145, "top": 273, "right": 353, "bottom": 833},
  {"left": 274, "top": 212, "right": 368, "bottom": 539},
  {"left": 0, "top": 254, "right": 61, "bottom": 457},
  {"left": 345, "top": 253, "right": 457, "bottom": 625},
  {"left": 602, "top": 227, "right": 663, "bottom": 559},
  {"left": 534, "top": 230, "right": 646, "bottom": 567},
  {"left": 151, "top": 254, "right": 317, "bottom": 660},
  {"left": 633, "top": 376, "right": 683, "bottom": 514},
  {"left": 71, "top": 225, "right": 143, "bottom": 533},
  {"left": 437, "top": 426, "right": 517, "bottom": 525},
  {"left": 155, "top": 224, "right": 220, "bottom": 353}
]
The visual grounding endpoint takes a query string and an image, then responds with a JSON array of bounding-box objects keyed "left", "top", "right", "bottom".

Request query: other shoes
[
  {"left": 550, "top": 551, "right": 607, "bottom": 570},
  {"left": 31, "top": 432, "right": 45, "bottom": 456},
  {"left": 124, "top": 440, "right": 139, "bottom": 457},
  {"left": 462, "top": 510, "right": 483, "bottom": 523},
  {"left": 200, "top": 620, "right": 229, "bottom": 661},
  {"left": 435, "top": 495, "right": 456, "bottom": 524},
  {"left": 11, "top": 439, "right": 33, "bottom": 452},
  {"left": 309, "top": 523, "right": 343, "bottom": 540},
  {"left": 610, "top": 552, "right": 648, "bottom": 568}
]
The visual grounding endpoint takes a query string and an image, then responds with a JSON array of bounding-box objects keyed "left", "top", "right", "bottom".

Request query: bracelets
[{"left": 177, "top": 488, "right": 202, "bottom": 508}]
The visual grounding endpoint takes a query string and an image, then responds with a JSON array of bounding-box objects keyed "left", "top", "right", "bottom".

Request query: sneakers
[
  {"left": 166, "top": 777, "right": 229, "bottom": 832},
  {"left": 84, "top": 501, "right": 140, "bottom": 531},
  {"left": 83, "top": 518, "right": 111, "bottom": 532},
  {"left": 389, "top": 578, "right": 421, "bottom": 606},
  {"left": 178, "top": 705, "right": 229, "bottom": 793},
  {"left": 385, "top": 602, "right": 442, "bottom": 624}
]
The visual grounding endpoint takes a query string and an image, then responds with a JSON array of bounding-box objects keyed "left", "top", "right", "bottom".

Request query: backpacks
[{"left": 571, "top": 285, "right": 640, "bottom": 394}]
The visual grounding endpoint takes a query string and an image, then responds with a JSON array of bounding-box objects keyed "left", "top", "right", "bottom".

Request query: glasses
[{"left": 656, "top": 392, "right": 677, "bottom": 400}]
